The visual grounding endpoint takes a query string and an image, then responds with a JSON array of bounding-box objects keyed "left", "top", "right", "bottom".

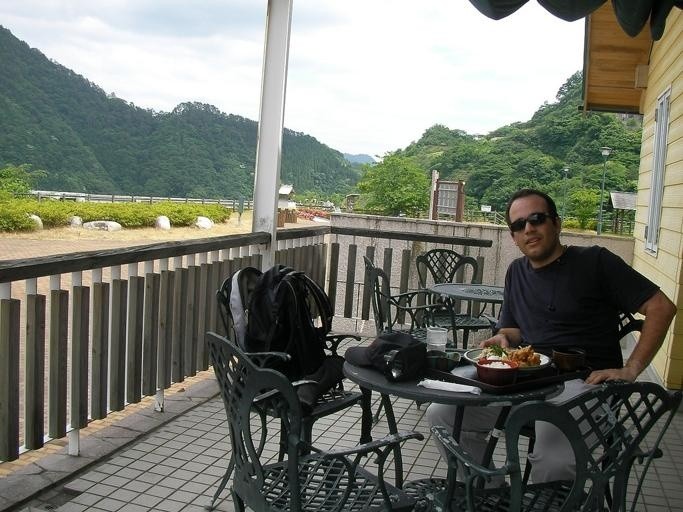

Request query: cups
[{"left": 426, "top": 326, "right": 448, "bottom": 353}]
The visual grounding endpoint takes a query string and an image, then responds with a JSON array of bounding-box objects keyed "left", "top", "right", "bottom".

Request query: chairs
[
  {"left": 416, "top": 249, "right": 498, "bottom": 350},
  {"left": 430, "top": 381, "right": 683, "bottom": 512},
  {"left": 205, "top": 331, "right": 425, "bottom": 512},
  {"left": 518, "top": 309, "right": 645, "bottom": 512},
  {"left": 363, "top": 256, "right": 457, "bottom": 420},
  {"left": 203, "top": 264, "right": 372, "bottom": 510}
]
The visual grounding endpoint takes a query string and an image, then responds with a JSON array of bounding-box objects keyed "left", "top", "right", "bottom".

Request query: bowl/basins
[
  {"left": 458, "top": 344, "right": 553, "bottom": 381},
  {"left": 551, "top": 346, "right": 585, "bottom": 373},
  {"left": 476, "top": 357, "right": 519, "bottom": 386}
]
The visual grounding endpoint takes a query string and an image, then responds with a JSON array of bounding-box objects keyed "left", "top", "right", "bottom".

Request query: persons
[{"left": 422, "top": 189, "right": 678, "bottom": 511}]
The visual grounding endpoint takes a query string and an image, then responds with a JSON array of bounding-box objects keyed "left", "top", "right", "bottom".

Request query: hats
[{"left": 346, "top": 330, "right": 426, "bottom": 369}]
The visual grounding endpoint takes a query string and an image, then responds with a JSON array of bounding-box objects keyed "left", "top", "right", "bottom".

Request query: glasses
[{"left": 508, "top": 211, "right": 553, "bottom": 232}]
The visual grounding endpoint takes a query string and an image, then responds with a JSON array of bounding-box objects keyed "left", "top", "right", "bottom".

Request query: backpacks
[{"left": 220, "top": 264, "right": 345, "bottom": 414}]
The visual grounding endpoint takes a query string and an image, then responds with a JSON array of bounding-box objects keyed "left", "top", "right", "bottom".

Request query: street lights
[
  {"left": 560, "top": 166, "right": 571, "bottom": 222},
  {"left": 594, "top": 146, "right": 613, "bottom": 235}
]
